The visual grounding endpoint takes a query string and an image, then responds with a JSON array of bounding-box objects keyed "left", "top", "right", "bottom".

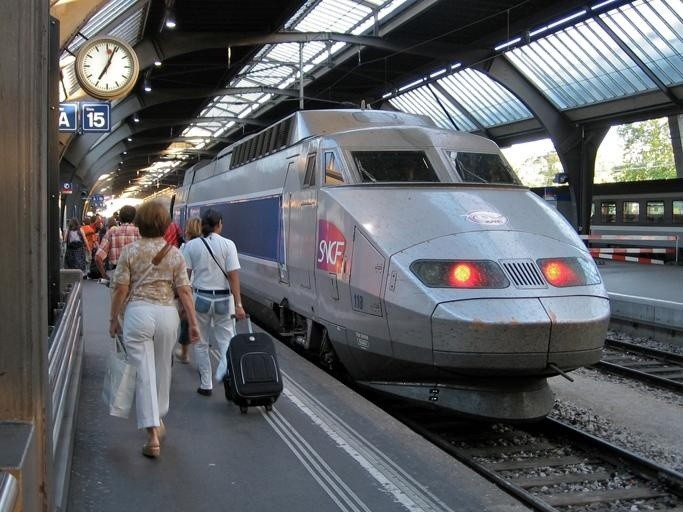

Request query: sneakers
[
  {"left": 196, "top": 386, "right": 212, "bottom": 396},
  {"left": 175, "top": 349, "right": 190, "bottom": 364}
]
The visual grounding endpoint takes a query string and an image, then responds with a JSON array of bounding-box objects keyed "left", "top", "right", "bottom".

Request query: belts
[{"left": 193, "top": 287, "right": 230, "bottom": 297}]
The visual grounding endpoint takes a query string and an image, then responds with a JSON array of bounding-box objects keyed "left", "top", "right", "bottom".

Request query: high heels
[{"left": 141, "top": 418, "right": 167, "bottom": 457}]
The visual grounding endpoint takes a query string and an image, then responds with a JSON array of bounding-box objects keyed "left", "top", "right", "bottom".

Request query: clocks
[{"left": 74, "top": 34, "right": 139, "bottom": 101}]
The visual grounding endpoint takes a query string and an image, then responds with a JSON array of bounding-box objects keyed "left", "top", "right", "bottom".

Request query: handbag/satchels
[{"left": 99, "top": 333, "right": 137, "bottom": 420}]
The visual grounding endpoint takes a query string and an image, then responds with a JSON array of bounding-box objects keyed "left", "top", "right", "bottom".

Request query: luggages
[
  {"left": 223, "top": 312, "right": 284, "bottom": 415},
  {"left": 86, "top": 247, "right": 106, "bottom": 281}
]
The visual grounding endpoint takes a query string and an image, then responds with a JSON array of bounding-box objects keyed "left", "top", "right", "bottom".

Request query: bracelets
[{"left": 235, "top": 304, "right": 242, "bottom": 307}]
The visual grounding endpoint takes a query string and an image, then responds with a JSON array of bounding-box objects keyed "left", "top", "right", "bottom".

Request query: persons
[
  {"left": 107, "top": 200, "right": 201, "bottom": 460},
  {"left": 177, "top": 205, "right": 245, "bottom": 397},
  {"left": 64, "top": 202, "right": 209, "bottom": 369}
]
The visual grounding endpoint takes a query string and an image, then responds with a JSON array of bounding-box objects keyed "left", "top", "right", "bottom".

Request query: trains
[{"left": 131, "top": 108, "right": 611, "bottom": 422}]
